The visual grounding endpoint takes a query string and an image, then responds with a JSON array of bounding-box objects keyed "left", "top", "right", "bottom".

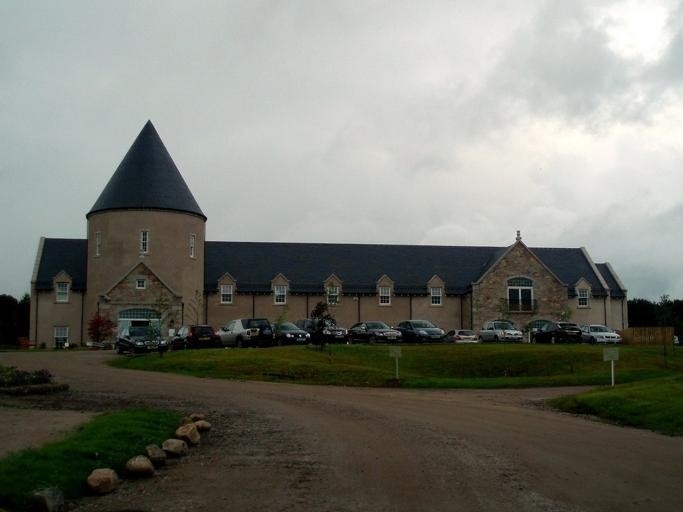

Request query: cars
[
  {"left": 392, "top": 320, "right": 446, "bottom": 343},
  {"left": 271, "top": 323, "right": 311, "bottom": 345},
  {"left": 346, "top": 321, "right": 404, "bottom": 343},
  {"left": 114, "top": 326, "right": 170, "bottom": 355},
  {"left": 581, "top": 324, "right": 622, "bottom": 344},
  {"left": 533, "top": 322, "right": 581, "bottom": 345},
  {"left": 441, "top": 329, "right": 479, "bottom": 343}
]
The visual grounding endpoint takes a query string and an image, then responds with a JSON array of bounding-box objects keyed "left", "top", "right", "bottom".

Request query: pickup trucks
[
  {"left": 216, "top": 317, "right": 277, "bottom": 348},
  {"left": 169, "top": 324, "right": 221, "bottom": 349},
  {"left": 478, "top": 321, "right": 523, "bottom": 342}
]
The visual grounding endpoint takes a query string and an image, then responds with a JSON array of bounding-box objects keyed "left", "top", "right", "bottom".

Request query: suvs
[{"left": 295, "top": 318, "right": 349, "bottom": 343}]
